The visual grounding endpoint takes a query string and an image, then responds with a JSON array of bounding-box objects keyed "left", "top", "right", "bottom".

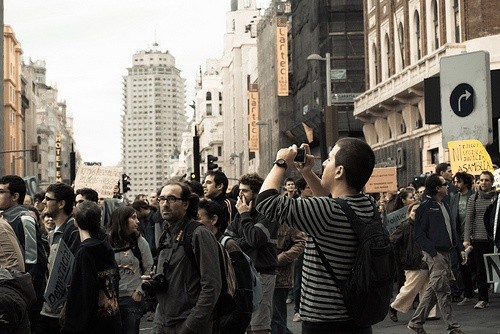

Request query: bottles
[{"left": 465, "top": 245, "right": 473, "bottom": 255}]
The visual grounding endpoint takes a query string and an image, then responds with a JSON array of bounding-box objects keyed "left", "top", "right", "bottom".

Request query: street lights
[
  {"left": 230, "top": 152, "right": 242, "bottom": 175},
  {"left": 256, "top": 119, "right": 272, "bottom": 172},
  {"left": 305, "top": 53, "right": 334, "bottom": 156}
]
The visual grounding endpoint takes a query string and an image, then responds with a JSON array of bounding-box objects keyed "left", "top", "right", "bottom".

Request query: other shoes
[
  {"left": 408, "top": 325, "right": 426, "bottom": 334},
  {"left": 286, "top": 298, "right": 292, "bottom": 304},
  {"left": 293, "top": 313, "right": 302, "bottom": 322},
  {"left": 457, "top": 297, "right": 477, "bottom": 305},
  {"left": 448, "top": 330, "right": 465, "bottom": 334},
  {"left": 474, "top": 300, "right": 489, "bottom": 308},
  {"left": 425, "top": 316, "right": 441, "bottom": 321},
  {"left": 389, "top": 304, "right": 398, "bottom": 322}
]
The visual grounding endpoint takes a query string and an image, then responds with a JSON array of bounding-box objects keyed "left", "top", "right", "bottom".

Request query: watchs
[{"left": 272, "top": 159, "right": 288, "bottom": 171}]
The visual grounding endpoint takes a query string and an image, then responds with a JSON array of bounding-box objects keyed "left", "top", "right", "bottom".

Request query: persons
[
  {"left": 254, "top": 137, "right": 383, "bottom": 334},
  {"left": 151, "top": 182, "right": 222, "bottom": 334},
  {"left": 0, "top": 162, "right": 500, "bottom": 334},
  {"left": 60, "top": 201, "right": 123, "bottom": 334},
  {"left": 406, "top": 174, "right": 468, "bottom": 334}
]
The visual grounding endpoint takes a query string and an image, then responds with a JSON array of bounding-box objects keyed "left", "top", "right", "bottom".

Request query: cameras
[
  {"left": 293, "top": 148, "right": 307, "bottom": 163},
  {"left": 139, "top": 274, "right": 167, "bottom": 295}
]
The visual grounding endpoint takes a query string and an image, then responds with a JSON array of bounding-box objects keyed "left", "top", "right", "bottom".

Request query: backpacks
[
  {"left": 311, "top": 196, "right": 397, "bottom": 327},
  {"left": 159, "top": 222, "right": 238, "bottom": 319},
  {"left": 222, "top": 235, "right": 264, "bottom": 312}
]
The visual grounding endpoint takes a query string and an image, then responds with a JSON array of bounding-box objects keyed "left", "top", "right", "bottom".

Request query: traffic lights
[
  {"left": 207, "top": 155, "right": 218, "bottom": 171},
  {"left": 191, "top": 173, "right": 195, "bottom": 180},
  {"left": 120, "top": 174, "right": 130, "bottom": 193}
]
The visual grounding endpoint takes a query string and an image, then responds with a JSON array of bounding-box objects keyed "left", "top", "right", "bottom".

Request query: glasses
[
  {"left": 0, "top": 189, "right": 9, "bottom": 193},
  {"left": 442, "top": 183, "right": 447, "bottom": 186},
  {"left": 74, "top": 200, "right": 84, "bottom": 206},
  {"left": 157, "top": 196, "right": 182, "bottom": 203},
  {"left": 46, "top": 195, "right": 56, "bottom": 201}
]
